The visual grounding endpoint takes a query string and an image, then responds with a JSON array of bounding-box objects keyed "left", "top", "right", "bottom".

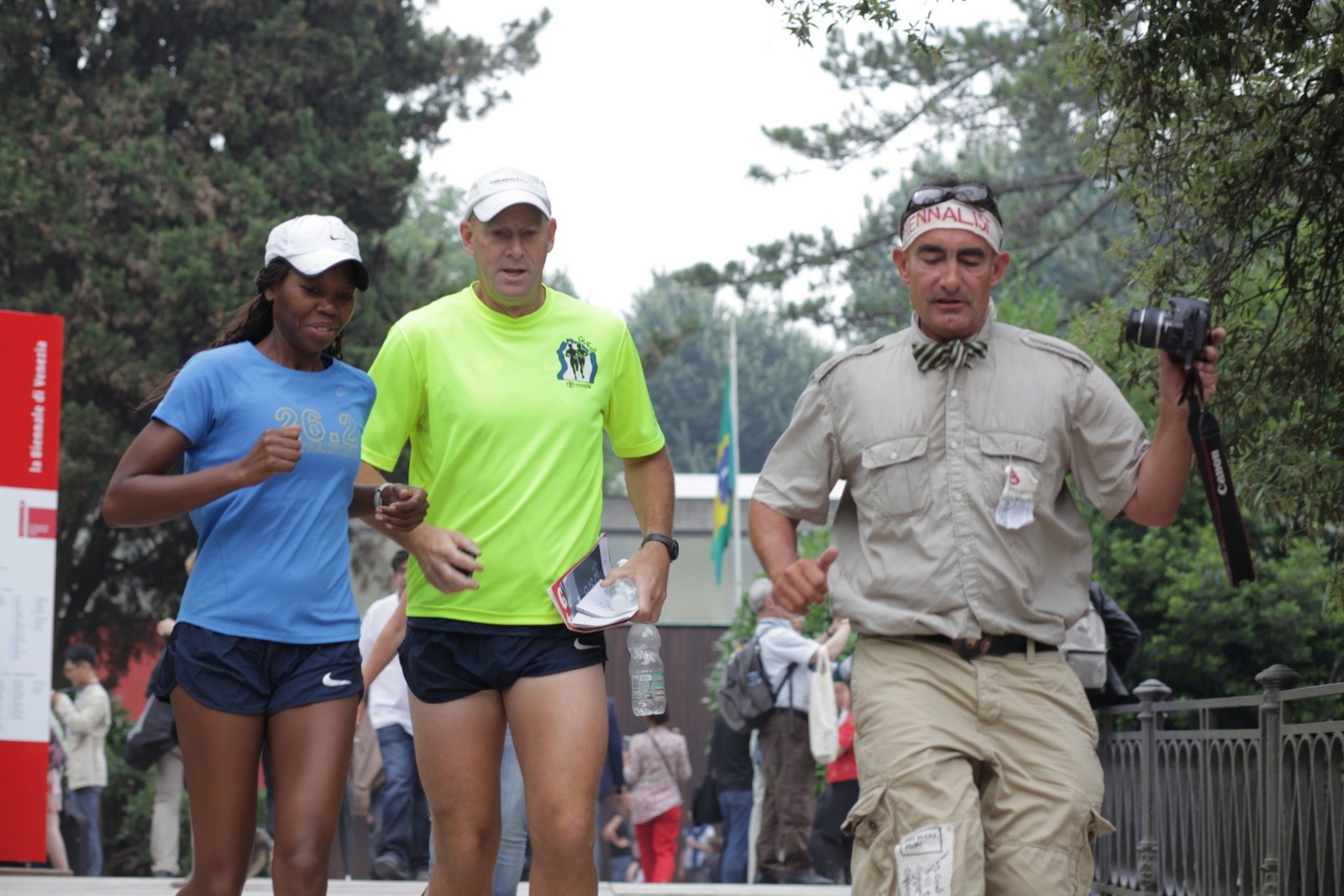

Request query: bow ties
[{"left": 914, "top": 338, "right": 990, "bottom": 373}]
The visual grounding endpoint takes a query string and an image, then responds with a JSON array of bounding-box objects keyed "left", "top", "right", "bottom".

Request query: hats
[
  {"left": 265, "top": 214, "right": 362, "bottom": 277},
  {"left": 464, "top": 166, "right": 552, "bottom": 222}
]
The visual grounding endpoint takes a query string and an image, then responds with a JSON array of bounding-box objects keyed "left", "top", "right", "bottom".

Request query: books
[{"left": 548, "top": 533, "right": 629, "bottom": 633}]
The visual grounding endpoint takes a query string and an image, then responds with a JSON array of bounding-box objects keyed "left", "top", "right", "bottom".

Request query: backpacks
[
  {"left": 1059, "top": 600, "right": 1106, "bottom": 688},
  {"left": 717, "top": 624, "right": 798, "bottom": 736}
]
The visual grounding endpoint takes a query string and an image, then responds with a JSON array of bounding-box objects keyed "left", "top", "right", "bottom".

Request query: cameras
[{"left": 1126, "top": 297, "right": 1213, "bottom": 372}]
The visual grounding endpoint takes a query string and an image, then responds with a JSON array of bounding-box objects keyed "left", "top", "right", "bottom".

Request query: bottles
[{"left": 626, "top": 622, "right": 666, "bottom": 717}]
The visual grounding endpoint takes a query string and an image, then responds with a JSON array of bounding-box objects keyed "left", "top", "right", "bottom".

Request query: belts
[{"left": 897, "top": 631, "right": 1057, "bottom": 659}]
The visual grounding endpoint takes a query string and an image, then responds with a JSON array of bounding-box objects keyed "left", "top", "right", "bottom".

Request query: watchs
[{"left": 640, "top": 533, "right": 679, "bottom": 561}]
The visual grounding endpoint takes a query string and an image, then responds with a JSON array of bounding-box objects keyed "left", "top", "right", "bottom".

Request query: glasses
[{"left": 905, "top": 182, "right": 991, "bottom": 212}]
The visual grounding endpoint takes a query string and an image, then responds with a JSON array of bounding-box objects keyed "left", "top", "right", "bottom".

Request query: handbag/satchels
[
  {"left": 691, "top": 775, "right": 724, "bottom": 827},
  {"left": 809, "top": 645, "right": 840, "bottom": 764}
]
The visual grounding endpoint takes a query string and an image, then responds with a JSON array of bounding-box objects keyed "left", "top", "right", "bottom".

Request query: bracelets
[{"left": 374, "top": 482, "right": 393, "bottom": 508}]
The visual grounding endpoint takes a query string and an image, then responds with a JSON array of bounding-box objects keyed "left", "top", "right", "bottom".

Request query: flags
[{"left": 711, "top": 364, "right": 734, "bottom": 587}]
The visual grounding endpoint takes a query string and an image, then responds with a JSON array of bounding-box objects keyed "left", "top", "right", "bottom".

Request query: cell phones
[{"left": 454, "top": 547, "right": 475, "bottom": 578}]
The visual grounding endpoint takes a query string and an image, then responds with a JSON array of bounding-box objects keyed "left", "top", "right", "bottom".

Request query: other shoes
[
  {"left": 785, "top": 869, "right": 833, "bottom": 884},
  {"left": 375, "top": 851, "right": 412, "bottom": 879},
  {"left": 754, "top": 869, "right": 778, "bottom": 883}
]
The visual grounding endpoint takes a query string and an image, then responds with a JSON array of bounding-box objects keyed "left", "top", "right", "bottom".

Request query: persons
[
  {"left": 749, "top": 172, "right": 1224, "bottom": 896},
  {"left": 52, "top": 645, "right": 113, "bottom": 874},
  {"left": 125, "top": 545, "right": 360, "bottom": 881},
  {"left": 43, "top": 737, "right": 70, "bottom": 870},
  {"left": 354, "top": 167, "right": 676, "bottom": 896},
  {"left": 602, "top": 797, "right": 718, "bottom": 883},
  {"left": 358, "top": 543, "right": 627, "bottom": 896},
  {"left": 101, "top": 214, "right": 427, "bottom": 896},
  {"left": 620, "top": 701, "right": 691, "bottom": 879},
  {"left": 1067, "top": 576, "right": 1144, "bottom": 703},
  {"left": 710, "top": 580, "right": 861, "bottom": 887}
]
